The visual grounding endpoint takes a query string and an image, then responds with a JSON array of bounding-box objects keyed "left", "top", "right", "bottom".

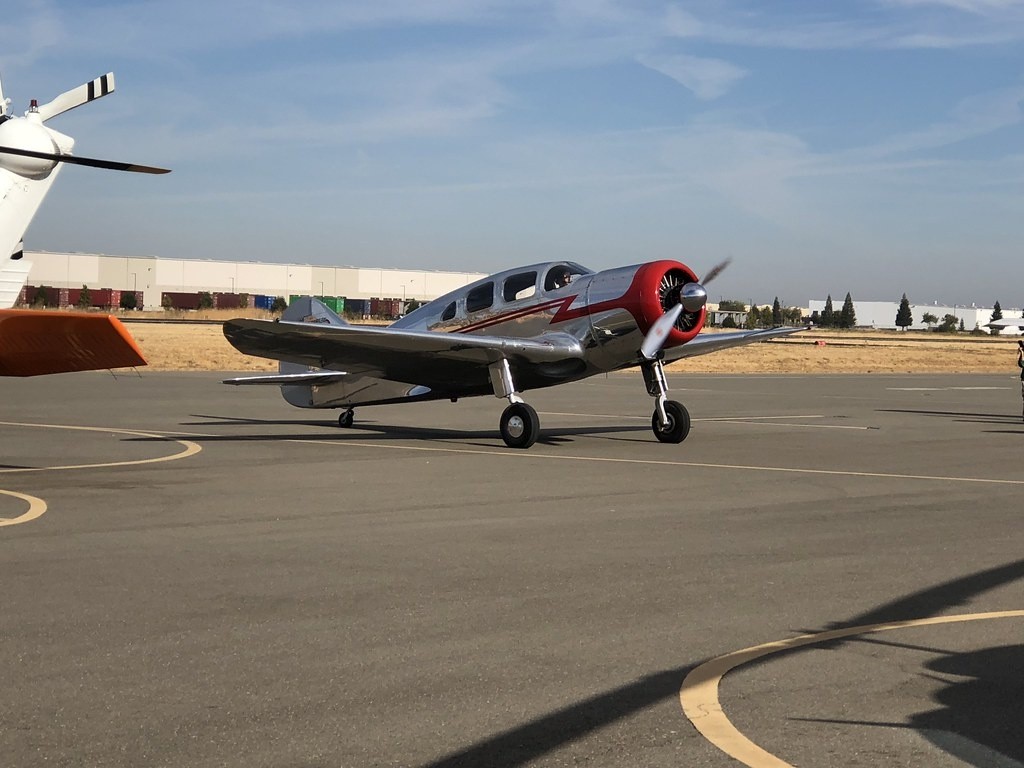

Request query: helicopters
[
  {"left": 0, "top": 72, "right": 173, "bottom": 302},
  {"left": 218, "top": 255, "right": 809, "bottom": 450}
]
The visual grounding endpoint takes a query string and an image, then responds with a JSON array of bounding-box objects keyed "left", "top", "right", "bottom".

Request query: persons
[{"left": 558, "top": 271, "right": 573, "bottom": 288}]
